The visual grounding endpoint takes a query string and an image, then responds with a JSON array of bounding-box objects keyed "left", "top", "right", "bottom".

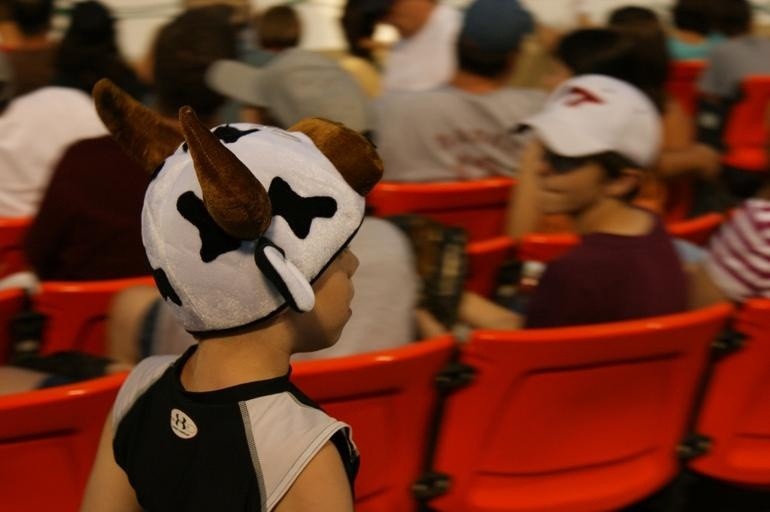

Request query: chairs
[
  {"left": 289, "top": 175, "right": 770, "bottom": 512},
  {"left": 0, "top": 216, "right": 156, "bottom": 512},
  {"left": 669, "top": 60, "right": 770, "bottom": 169}
]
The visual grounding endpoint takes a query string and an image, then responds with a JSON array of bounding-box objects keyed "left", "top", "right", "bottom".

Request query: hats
[
  {"left": 203, "top": 48, "right": 383, "bottom": 135},
  {"left": 91, "top": 77, "right": 385, "bottom": 343},
  {"left": 509, "top": 72, "right": 666, "bottom": 174}
]
